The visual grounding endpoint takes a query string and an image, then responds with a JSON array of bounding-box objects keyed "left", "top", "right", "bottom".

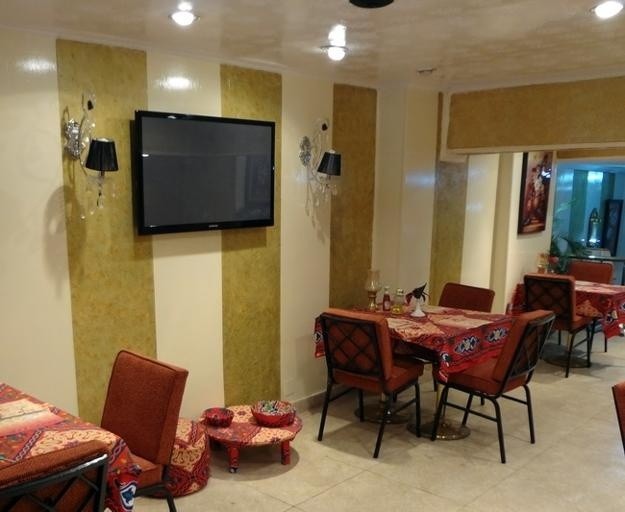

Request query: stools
[{"left": 139, "top": 416, "right": 211, "bottom": 499}]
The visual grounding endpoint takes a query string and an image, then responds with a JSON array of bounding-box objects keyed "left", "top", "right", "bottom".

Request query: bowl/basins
[
  {"left": 201, "top": 408, "right": 234, "bottom": 425},
  {"left": 251, "top": 399, "right": 296, "bottom": 427}
]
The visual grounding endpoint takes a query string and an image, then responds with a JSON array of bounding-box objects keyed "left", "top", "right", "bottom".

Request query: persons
[{"left": 522, "top": 168, "right": 546, "bottom": 226}]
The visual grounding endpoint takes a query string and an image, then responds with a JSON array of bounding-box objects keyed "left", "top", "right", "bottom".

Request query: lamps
[
  {"left": 297, "top": 117, "right": 343, "bottom": 226},
  {"left": 61, "top": 92, "right": 119, "bottom": 218}
]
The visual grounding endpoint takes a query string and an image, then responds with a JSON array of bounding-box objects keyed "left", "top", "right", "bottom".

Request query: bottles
[
  {"left": 389, "top": 288, "right": 405, "bottom": 316},
  {"left": 383, "top": 285, "right": 391, "bottom": 311}
]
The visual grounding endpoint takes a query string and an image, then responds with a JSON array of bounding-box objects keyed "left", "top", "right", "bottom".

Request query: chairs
[
  {"left": 433, "top": 281, "right": 498, "bottom": 421},
  {"left": 612, "top": 378, "right": 625, "bottom": 452},
  {"left": 99, "top": 348, "right": 188, "bottom": 511},
  {"left": 430, "top": 311, "right": 556, "bottom": 463},
  {"left": 319, "top": 307, "right": 424, "bottom": 457},
  {"left": 560, "top": 259, "right": 614, "bottom": 355},
  {"left": 524, "top": 272, "right": 596, "bottom": 377}
]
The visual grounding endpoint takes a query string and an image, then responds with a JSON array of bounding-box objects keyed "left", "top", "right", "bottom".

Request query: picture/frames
[{"left": 517, "top": 152, "right": 553, "bottom": 236}]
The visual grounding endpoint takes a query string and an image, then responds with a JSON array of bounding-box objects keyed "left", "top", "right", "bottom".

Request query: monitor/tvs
[{"left": 135, "top": 110, "right": 275, "bottom": 236}]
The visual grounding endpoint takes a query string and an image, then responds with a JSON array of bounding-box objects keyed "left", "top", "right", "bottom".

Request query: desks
[
  {"left": 588, "top": 248, "right": 611, "bottom": 259},
  {"left": 198, "top": 400, "right": 303, "bottom": 475},
  {"left": 0, "top": 380, "right": 140, "bottom": 511},
  {"left": 313, "top": 298, "right": 514, "bottom": 441},
  {"left": 505, "top": 277, "right": 625, "bottom": 368}
]
